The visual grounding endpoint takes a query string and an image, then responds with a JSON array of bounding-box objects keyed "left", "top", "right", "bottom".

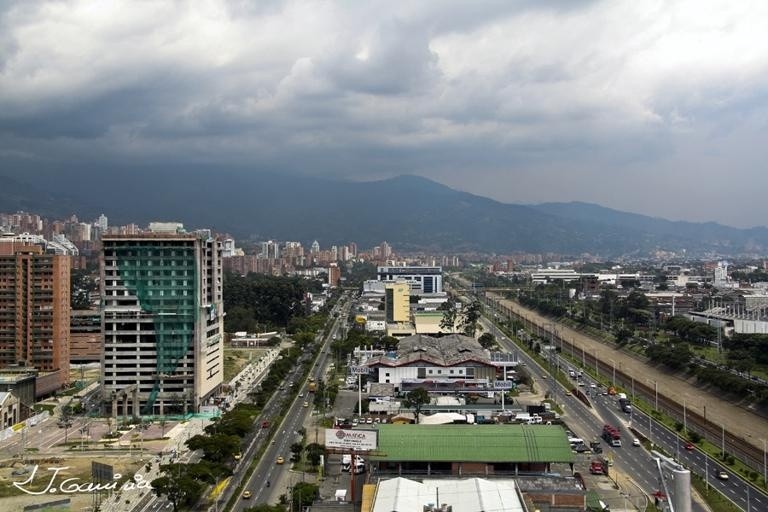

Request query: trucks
[{"left": 319, "top": 454, "right": 365, "bottom": 470}]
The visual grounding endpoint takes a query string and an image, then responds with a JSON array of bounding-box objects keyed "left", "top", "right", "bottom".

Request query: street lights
[
  {"left": 209, "top": 472, "right": 219, "bottom": 511},
  {"left": 484, "top": 296, "right": 766, "bottom": 510}
]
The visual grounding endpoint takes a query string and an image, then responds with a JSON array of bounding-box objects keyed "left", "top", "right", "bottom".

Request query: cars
[
  {"left": 352, "top": 417, "right": 386, "bottom": 428},
  {"left": 332, "top": 333, "right": 336, "bottom": 340},
  {"left": 234, "top": 452, "right": 243, "bottom": 460},
  {"left": 349, "top": 462, "right": 365, "bottom": 474},
  {"left": 332, "top": 290, "right": 358, "bottom": 321},
  {"left": 275, "top": 350, "right": 328, "bottom": 409},
  {"left": 242, "top": 489, "right": 251, "bottom": 499},
  {"left": 260, "top": 420, "right": 269, "bottom": 428},
  {"left": 276, "top": 455, "right": 284, "bottom": 464},
  {"left": 56, "top": 419, "right": 74, "bottom": 429}
]
